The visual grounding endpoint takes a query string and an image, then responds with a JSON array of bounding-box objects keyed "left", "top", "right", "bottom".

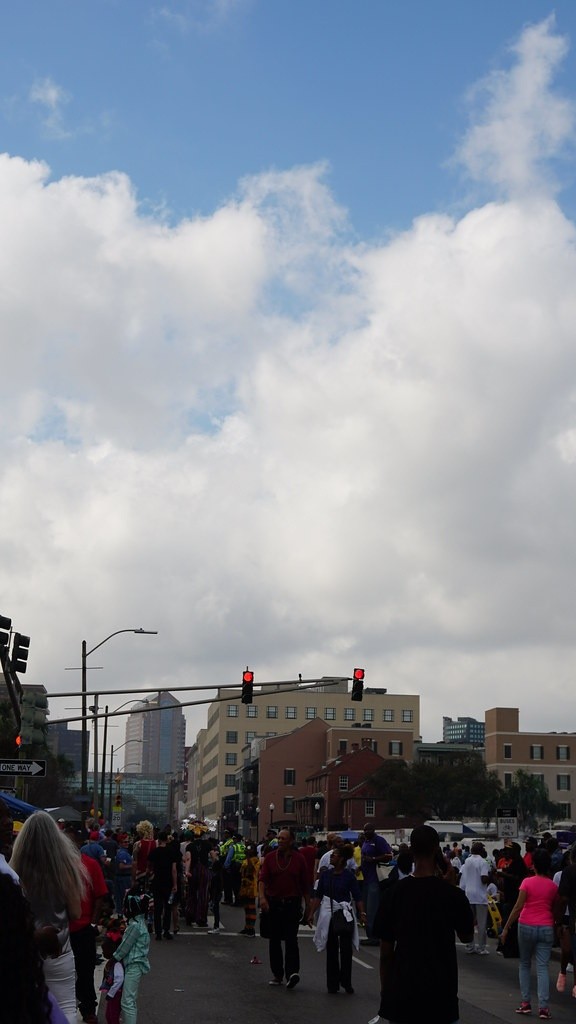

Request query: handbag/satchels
[
  {"left": 259, "top": 900, "right": 303, "bottom": 940},
  {"left": 333, "top": 911, "right": 354, "bottom": 936},
  {"left": 188, "top": 865, "right": 212, "bottom": 888}
]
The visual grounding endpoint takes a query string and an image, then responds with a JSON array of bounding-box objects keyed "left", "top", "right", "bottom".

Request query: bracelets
[{"left": 360, "top": 912, "right": 366, "bottom": 915}]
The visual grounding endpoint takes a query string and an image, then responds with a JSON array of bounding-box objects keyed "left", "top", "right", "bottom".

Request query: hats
[
  {"left": 265, "top": 829, "right": 277, "bottom": 837},
  {"left": 522, "top": 837, "right": 538, "bottom": 844},
  {"left": 232, "top": 833, "right": 242, "bottom": 839},
  {"left": 505, "top": 842, "right": 522, "bottom": 849}
]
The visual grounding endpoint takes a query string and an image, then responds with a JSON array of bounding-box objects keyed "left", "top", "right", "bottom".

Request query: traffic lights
[
  {"left": 114, "top": 794, "right": 122, "bottom": 813},
  {"left": 0, "top": 616, "right": 11, "bottom": 662},
  {"left": 11, "top": 632, "right": 31, "bottom": 675},
  {"left": 13, "top": 735, "right": 24, "bottom": 758},
  {"left": 241, "top": 671, "right": 254, "bottom": 705},
  {"left": 351, "top": 668, "right": 365, "bottom": 701}
]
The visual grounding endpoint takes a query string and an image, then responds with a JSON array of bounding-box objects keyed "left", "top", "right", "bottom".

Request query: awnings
[{"left": 0, "top": 792, "right": 46, "bottom": 817}]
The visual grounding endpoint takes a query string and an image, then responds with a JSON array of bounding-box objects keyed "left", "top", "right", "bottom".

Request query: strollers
[
  {"left": 120, "top": 874, "right": 154, "bottom": 934},
  {"left": 486, "top": 891, "right": 500, "bottom": 938}
]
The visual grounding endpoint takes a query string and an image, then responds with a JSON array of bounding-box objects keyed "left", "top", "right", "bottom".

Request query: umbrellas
[{"left": 44, "top": 805, "right": 84, "bottom": 822}]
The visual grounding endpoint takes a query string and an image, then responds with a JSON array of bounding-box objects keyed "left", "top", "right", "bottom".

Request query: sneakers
[
  {"left": 556, "top": 970, "right": 566, "bottom": 992},
  {"left": 572, "top": 985, "right": 576, "bottom": 998},
  {"left": 539, "top": 1008, "right": 552, "bottom": 1018},
  {"left": 516, "top": 1002, "right": 532, "bottom": 1013},
  {"left": 286, "top": 973, "right": 300, "bottom": 988},
  {"left": 269, "top": 979, "right": 283, "bottom": 984}
]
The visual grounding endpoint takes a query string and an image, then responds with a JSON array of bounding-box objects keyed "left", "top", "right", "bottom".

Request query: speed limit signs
[{"left": 112, "top": 814, "right": 122, "bottom": 826}]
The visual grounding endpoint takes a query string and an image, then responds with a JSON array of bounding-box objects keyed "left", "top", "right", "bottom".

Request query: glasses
[
  {"left": 330, "top": 854, "right": 339, "bottom": 858},
  {"left": 123, "top": 841, "right": 130, "bottom": 843}
]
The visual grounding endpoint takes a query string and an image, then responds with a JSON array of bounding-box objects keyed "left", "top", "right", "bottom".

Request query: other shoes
[
  {"left": 341, "top": 983, "right": 353, "bottom": 993},
  {"left": 237, "top": 927, "right": 255, "bottom": 937},
  {"left": 329, "top": 986, "right": 339, "bottom": 993},
  {"left": 478, "top": 949, "right": 490, "bottom": 954},
  {"left": 96, "top": 954, "right": 102, "bottom": 957},
  {"left": 147, "top": 901, "right": 236, "bottom": 942},
  {"left": 466, "top": 948, "right": 474, "bottom": 953}
]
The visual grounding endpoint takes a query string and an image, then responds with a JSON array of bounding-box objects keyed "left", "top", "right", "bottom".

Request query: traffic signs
[{"left": 0, "top": 759, "right": 47, "bottom": 778}]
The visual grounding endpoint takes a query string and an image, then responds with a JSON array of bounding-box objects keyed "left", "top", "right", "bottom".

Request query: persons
[
  {"left": 0, "top": 798, "right": 60, "bottom": 1024},
  {"left": 238, "top": 843, "right": 260, "bottom": 937},
  {"left": 358, "top": 822, "right": 393, "bottom": 946},
  {"left": 146, "top": 831, "right": 177, "bottom": 942},
  {"left": 465, "top": 843, "right": 491, "bottom": 955},
  {"left": 0, "top": 809, "right": 94, "bottom": 1024},
  {"left": 107, "top": 884, "right": 152, "bottom": 1024},
  {"left": 55, "top": 814, "right": 576, "bottom": 926},
  {"left": 306, "top": 844, "right": 368, "bottom": 995},
  {"left": 80, "top": 829, "right": 111, "bottom": 867},
  {"left": 501, "top": 848, "right": 559, "bottom": 1019},
  {"left": 555, "top": 846, "right": 576, "bottom": 999},
  {"left": 371, "top": 826, "right": 474, "bottom": 1024},
  {"left": 207, "top": 850, "right": 225, "bottom": 934},
  {"left": 65, "top": 851, "right": 109, "bottom": 1024},
  {"left": 99, "top": 927, "right": 124, "bottom": 1024},
  {"left": 257, "top": 829, "right": 311, "bottom": 989}
]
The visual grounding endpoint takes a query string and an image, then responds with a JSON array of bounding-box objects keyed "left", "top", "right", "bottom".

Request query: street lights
[
  {"left": 109, "top": 739, "right": 149, "bottom": 826},
  {"left": 223, "top": 816, "right": 227, "bottom": 829},
  {"left": 118, "top": 763, "right": 141, "bottom": 795},
  {"left": 270, "top": 803, "right": 274, "bottom": 829},
  {"left": 256, "top": 808, "right": 260, "bottom": 843},
  {"left": 101, "top": 699, "right": 149, "bottom": 812},
  {"left": 235, "top": 811, "right": 239, "bottom": 834},
  {"left": 315, "top": 803, "right": 320, "bottom": 832},
  {"left": 81, "top": 629, "right": 158, "bottom": 818}
]
